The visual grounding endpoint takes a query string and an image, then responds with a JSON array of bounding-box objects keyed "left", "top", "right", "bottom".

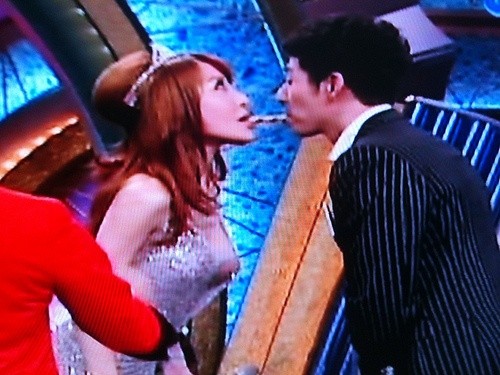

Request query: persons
[
  {"left": 46, "top": 49, "right": 256, "bottom": 375},
  {"left": 0, "top": 187, "right": 182, "bottom": 375},
  {"left": 275, "top": 12, "right": 500, "bottom": 375}
]
